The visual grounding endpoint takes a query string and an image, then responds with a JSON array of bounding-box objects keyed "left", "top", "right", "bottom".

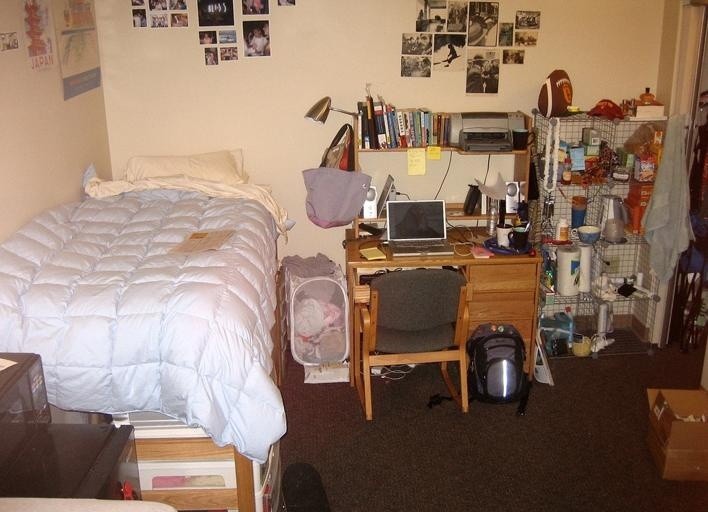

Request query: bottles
[
  {"left": 563, "top": 306, "right": 575, "bottom": 349},
  {"left": 556, "top": 218, "right": 568, "bottom": 241},
  {"left": 485, "top": 202, "right": 498, "bottom": 236},
  {"left": 639, "top": 85, "right": 655, "bottom": 105}
]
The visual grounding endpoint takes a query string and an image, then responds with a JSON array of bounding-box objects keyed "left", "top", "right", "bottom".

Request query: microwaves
[{"left": 1, "top": 351, "right": 53, "bottom": 484}]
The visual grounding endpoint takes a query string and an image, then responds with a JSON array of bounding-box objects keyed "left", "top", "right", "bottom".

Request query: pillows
[{"left": 127, "top": 147, "right": 246, "bottom": 184}]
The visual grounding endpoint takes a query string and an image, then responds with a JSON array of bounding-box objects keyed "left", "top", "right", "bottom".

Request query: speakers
[
  {"left": 505, "top": 182, "right": 519, "bottom": 214},
  {"left": 363, "top": 186, "right": 378, "bottom": 219},
  {"left": 520, "top": 181, "right": 526, "bottom": 203}
]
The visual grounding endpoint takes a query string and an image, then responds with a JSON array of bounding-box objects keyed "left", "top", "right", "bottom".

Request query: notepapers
[{"left": 359, "top": 247, "right": 386, "bottom": 260}]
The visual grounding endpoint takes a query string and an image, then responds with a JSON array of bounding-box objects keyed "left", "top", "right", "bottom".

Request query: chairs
[{"left": 353, "top": 268, "right": 472, "bottom": 421}]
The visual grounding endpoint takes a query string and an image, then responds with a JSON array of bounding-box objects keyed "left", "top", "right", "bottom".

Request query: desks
[{"left": 339, "top": 231, "right": 541, "bottom": 390}]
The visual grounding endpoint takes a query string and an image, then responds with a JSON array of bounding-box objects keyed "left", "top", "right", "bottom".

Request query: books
[
  {"left": 357, "top": 95, "right": 451, "bottom": 150},
  {"left": 624, "top": 98, "right": 665, "bottom": 118}
]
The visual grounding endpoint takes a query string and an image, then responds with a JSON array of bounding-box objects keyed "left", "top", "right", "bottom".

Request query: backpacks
[{"left": 426, "top": 323, "right": 533, "bottom": 418}]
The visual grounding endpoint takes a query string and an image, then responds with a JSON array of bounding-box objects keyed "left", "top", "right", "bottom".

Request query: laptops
[{"left": 386, "top": 199, "right": 454, "bottom": 257}]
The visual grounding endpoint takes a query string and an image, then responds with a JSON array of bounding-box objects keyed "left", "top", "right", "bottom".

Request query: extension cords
[{"left": 371, "top": 366, "right": 385, "bottom": 374}]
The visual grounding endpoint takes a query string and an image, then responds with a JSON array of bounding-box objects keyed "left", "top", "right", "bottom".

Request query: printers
[{"left": 448, "top": 112, "right": 526, "bottom": 152}]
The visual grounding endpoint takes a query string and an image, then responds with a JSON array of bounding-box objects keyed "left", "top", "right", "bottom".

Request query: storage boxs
[{"left": 648, "top": 388, "right": 708, "bottom": 481}]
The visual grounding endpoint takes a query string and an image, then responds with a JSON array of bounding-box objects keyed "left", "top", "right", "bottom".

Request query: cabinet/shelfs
[
  {"left": 350, "top": 111, "right": 535, "bottom": 238},
  {"left": 532, "top": 105, "right": 668, "bottom": 360}
]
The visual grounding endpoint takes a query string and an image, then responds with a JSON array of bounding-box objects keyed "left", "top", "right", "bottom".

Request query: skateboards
[{"left": 281, "top": 463, "right": 330, "bottom": 511}]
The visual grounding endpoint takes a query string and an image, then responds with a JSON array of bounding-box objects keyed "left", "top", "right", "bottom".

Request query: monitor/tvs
[{"left": 0, "top": 352, "right": 51, "bottom": 497}]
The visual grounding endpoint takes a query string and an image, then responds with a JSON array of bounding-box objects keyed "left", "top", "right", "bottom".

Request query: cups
[
  {"left": 496, "top": 224, "right": 512, "bottom": 249},
  {"left": 603, "top": 218, "right": 625, "bottom": 243},
  {"left": 569, "top": 225, "right": 601, "bottom": 244},
  {"left": 571, "top": 196, "right": 586, "bottom": 227},
  {"left": 507, "top": 225, "right": 530, "bottom": 250}
]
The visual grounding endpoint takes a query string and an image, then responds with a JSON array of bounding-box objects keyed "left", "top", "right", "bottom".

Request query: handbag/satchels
[{"left": 302, "top": 123, "right": 372, "bottom": 230}]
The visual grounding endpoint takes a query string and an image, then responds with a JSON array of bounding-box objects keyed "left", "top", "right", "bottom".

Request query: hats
[{"left": 587, "top": 100, "right": 624, "bottom": 122}]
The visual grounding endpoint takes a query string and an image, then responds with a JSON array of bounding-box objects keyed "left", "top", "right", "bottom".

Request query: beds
[{"left": 2, "top": 149, "right": 290, "bottom": 512}]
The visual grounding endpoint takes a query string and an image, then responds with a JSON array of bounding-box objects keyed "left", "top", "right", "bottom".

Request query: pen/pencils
[{"left": 524, "top": 221, "right": 530, "bottom": 232}]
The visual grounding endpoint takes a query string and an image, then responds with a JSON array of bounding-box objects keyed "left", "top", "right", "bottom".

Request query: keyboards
[{"left": 359, "top": 273, "right": 385, "bottom": 285}]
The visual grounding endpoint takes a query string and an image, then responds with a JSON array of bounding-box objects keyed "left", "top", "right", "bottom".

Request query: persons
[
  {"left": 130, "top": 0, "right": 294, "bottom": 64},
  {"left": 400, "top": 5, "right": 537, "bottom": 92}
]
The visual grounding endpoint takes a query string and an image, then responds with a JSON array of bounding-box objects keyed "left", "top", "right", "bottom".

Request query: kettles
[{"left": 596, "top": 194, "right": 628, "bottom": 240}]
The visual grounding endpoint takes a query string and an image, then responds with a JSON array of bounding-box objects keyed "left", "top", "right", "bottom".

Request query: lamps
[{"left": 304, "top": 96, "right": 360, "bottom": 126}]
